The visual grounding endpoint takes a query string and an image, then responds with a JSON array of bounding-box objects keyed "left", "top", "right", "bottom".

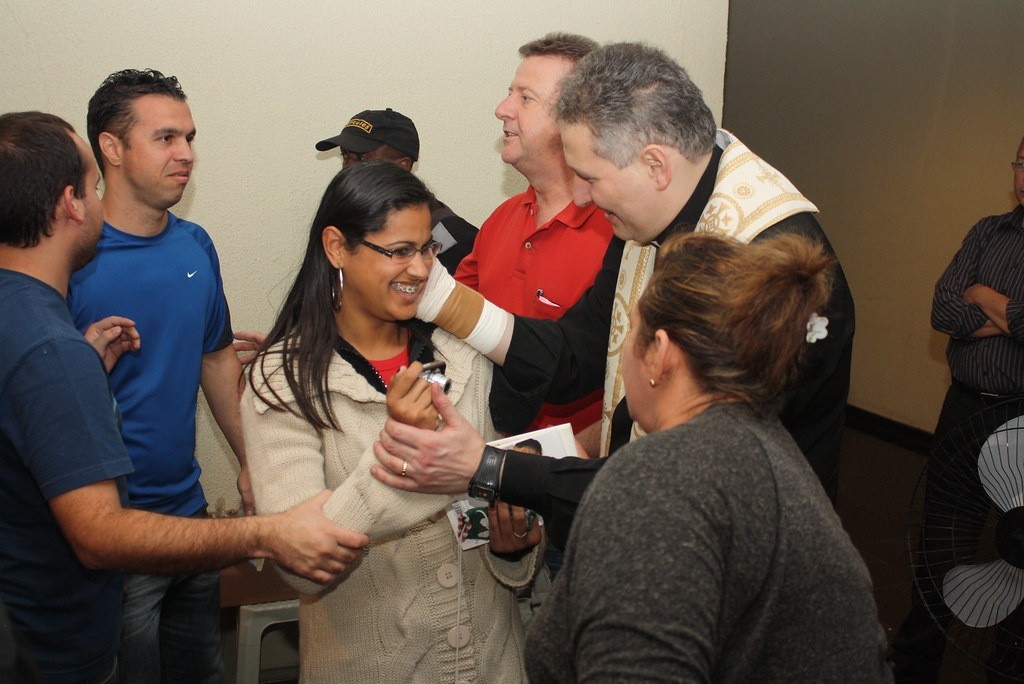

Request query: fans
[{"left": 905, "top": 397, "right": 1024, "bottom": 683}]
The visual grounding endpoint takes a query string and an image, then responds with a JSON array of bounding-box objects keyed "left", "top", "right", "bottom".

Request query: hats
[{"left": 315, "top": 108, "right": 420, "bottom": 162}]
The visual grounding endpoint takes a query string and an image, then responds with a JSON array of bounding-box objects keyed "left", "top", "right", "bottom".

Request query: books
[{"left": 444, "top": 422, "right": 579, "bottom": 551}]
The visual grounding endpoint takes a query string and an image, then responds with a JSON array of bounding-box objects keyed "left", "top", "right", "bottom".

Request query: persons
[
  {"left": 315, "top": 107, "right": 480, "bottom": 359},
  {"left": 886, "top": 137, "right": 1024, "bottom": 684},
  {"left": 0, "top": 110, "right": 369, "bottom": 684},
  {"left": 368, "top": 43, "right": 856, "bottom": 553},
  {"left": 239, "top": 158, "right": 545, "bottom": 684},
  {"left": 513, "top": 439, "right": 542, "bottom": 455},
  {"left": 232, "top": 31, "right": 605, "bottom": 597},
  {"left": 522, "top": 231, "right": 895, "bottom": 684},
  {"left": 65, "top": 68, "right": 254, "bottom": 684}
]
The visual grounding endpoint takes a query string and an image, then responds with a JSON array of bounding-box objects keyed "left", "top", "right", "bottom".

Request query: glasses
[{"left": 357, "top": 238, "right": 443, "bottom": 264}]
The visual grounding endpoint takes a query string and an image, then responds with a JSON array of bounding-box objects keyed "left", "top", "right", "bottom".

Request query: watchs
[{"left": 467, "top": 445, "right": 505, "bottom": 503}]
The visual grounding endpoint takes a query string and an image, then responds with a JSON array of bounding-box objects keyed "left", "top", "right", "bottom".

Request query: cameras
[{"left": 418, "top": 360, "right": 451, "bottom": 394}]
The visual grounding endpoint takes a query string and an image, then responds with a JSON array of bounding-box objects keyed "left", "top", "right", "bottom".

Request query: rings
[
  {"left": 402, "top": 461, "right": 407, "bottom": 476},
  {"left": 514, "top": 532, "right": 527, "bottom": 539}
]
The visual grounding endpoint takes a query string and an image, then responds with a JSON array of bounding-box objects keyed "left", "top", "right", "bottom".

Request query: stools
[{"left": 234, "top": 599, "right": 300, "bottom": 684}]
